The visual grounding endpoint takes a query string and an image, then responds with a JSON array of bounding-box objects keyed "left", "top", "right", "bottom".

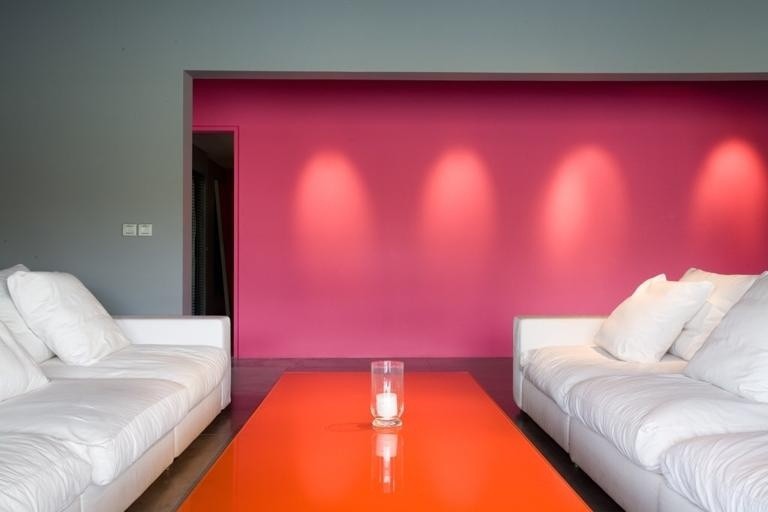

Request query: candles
[{"left": 375, "top": 393, "right": 397, "bottom": 417}]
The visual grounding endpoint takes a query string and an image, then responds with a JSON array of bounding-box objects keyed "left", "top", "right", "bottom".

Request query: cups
[{"left": 369, "top": 359, "right": 405, "bottom": 429}]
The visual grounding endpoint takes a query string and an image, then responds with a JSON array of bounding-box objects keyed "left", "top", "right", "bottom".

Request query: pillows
[
  {"left": 595, "top": 264, "right": 768, "bottom": 406},
  {"left": 0, "top": 264, "right": 130, "bottom": 400}
]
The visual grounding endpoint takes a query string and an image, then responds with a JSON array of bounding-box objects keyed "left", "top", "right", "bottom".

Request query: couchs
[
  {"left": 514, "top": 316, "right": 768, "bottom": 510},
  {"left": 1, "top": 312, "right": 234, "bottom": 510}
]
